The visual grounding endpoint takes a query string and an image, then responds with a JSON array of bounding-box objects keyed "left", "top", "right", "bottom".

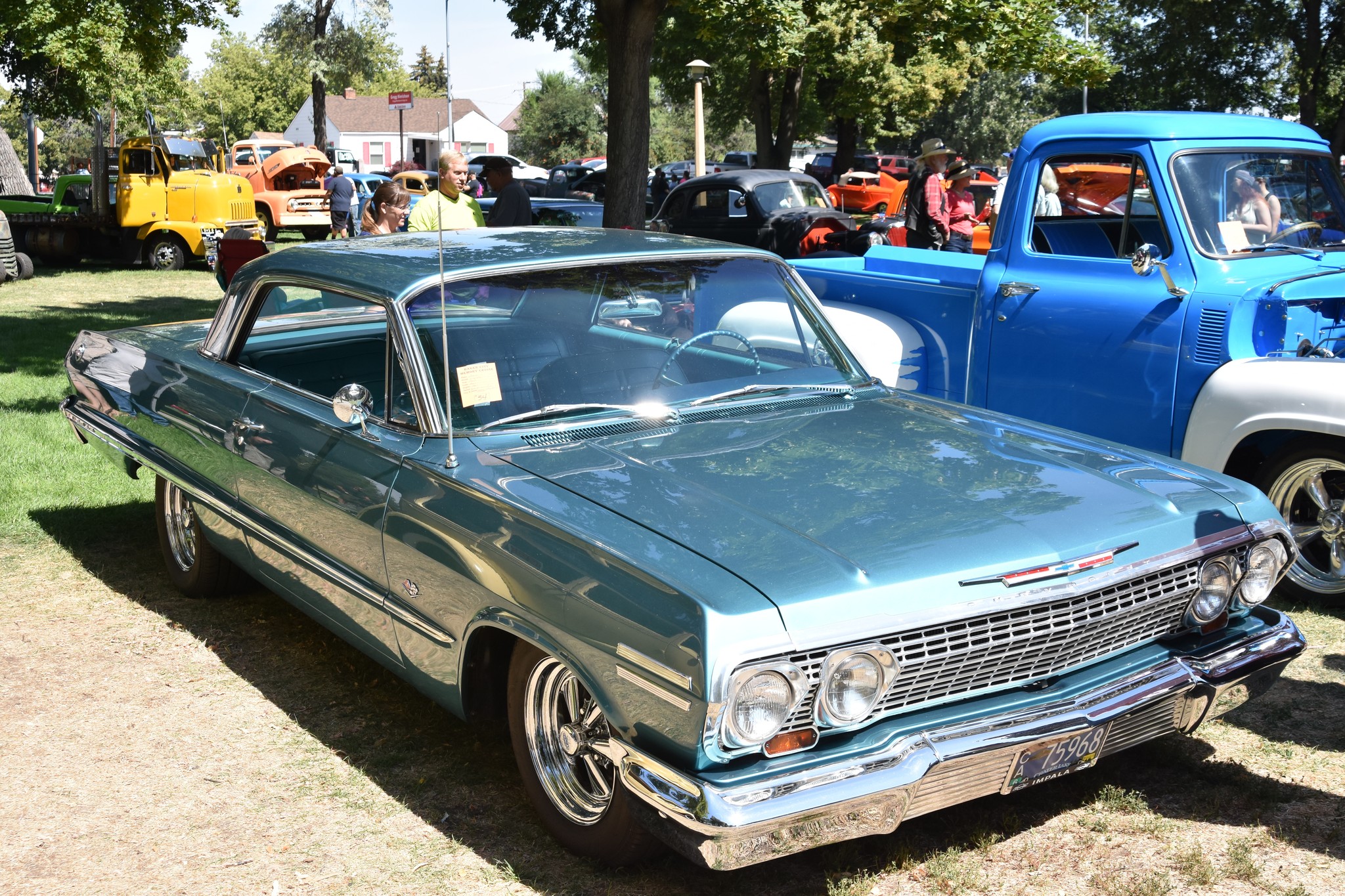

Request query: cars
[
  {"left": 805, "top": 148, "right": 1148, "bottom": 256},
  {"left": 392, "top": 170, "right": 439, "bottom": 195},
  {"left": 68, "top": 227, "right": 1312, "bottom": 865},
  {"left": 464, "top": 153, "right": 555, "bottom": 192},
  {"left": 548, "top": 150, "right": 763, "bottom": 216},
  {"left": 639, "top": 166, "right": 867, "bottom": 263},
  {"left": 474, "top": 195, "right": 606, "bottom": 230},
  {"left": 323, "top": 173, "right": 426, "bottom": 232},
  {"left": 701, "top": 112, "right": 1344, "bottom": 582}
]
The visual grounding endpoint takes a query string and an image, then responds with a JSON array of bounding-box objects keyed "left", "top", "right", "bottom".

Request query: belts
[{"left": 950, "top": 231, "right": 973, "bottom": 240}]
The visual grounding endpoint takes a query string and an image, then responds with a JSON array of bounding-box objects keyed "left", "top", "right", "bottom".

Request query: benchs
[
  {"left": 1033, "top": 219, "right": 1169, "bottom": 258},
  {"left": 252, "top": 324, "right": 576, "bottom": 424}
]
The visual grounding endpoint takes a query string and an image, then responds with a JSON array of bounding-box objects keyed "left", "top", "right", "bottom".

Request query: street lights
[{"left": 685, "top": 58, "right": 713, "bottom": 207}]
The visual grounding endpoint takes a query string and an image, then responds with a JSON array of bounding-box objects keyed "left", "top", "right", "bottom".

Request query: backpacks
[{"left": 477, "top": 182, "right": 483, "bottom": 198}]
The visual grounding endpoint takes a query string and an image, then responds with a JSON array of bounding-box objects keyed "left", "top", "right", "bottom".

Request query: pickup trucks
[{"left": 1, "top": 118, "right": 358, "bottom": 272}]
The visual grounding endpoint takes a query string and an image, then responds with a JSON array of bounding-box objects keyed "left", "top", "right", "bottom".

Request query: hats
[
  {"left": 1235, "top": 170, "right": 1255, "bottom": 186},
  {"left": 1003, "top": 148, "right": 1017, "bottom": 159},
  {"left": 332, "top": 166, "right": 343, "bottom": 173},
  {"left": 914, "top": 138, "right": 957, "bottom": 160},
  {"left": 478, "top": 157, "right": 513, "bottom": 177},
  {"left": 945, "top": 160, "right": 981, "bottom": 183},
  {"left": 467, "top": 170, "right": 476, "bottom": 175}
]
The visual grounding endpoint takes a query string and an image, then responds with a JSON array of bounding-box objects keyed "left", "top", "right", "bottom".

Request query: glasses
[
  {"left": 482, "top": 166, "right": 499, "bottom": 173},
  {"left": 384, "top": 203, "right": 410, "bottom": 212}
]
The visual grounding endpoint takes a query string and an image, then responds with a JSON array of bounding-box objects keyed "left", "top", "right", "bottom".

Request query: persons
[
  {"left": 325, "top": 172, "right": 331, "bottom": 181},
  {"left": 72, "top": 163, "right": 90, "bottom": 199},
  {"left": 945, "top": 160, "right": 980, "bottom": 254},
  {"left": 651, "top": 168, "right": 690, "bottom": 220},
  {"left": 213, "top": 227, "right": 323, "bottom": 317},
  {"left": 478, "top": 157, "right": 532, "bottom": 227},
  {"left": 989, "top": 149, "right": 1063, "bottom": 243},
  {"left": 407, "top": 150, "right": 490, "bottom": 307},
  {"left": 463, "top": 170, "right": 483, "bottom": 199},
  {"left": 904, "top": 138, "right": 957, "bottom": 251},
  {"left": 358, "top": 180, "right": 412, "bottom": 236},
  {"left": 1252, "top": 173, "right": 1281, "bottom": 241},
  {"left": 345, "top": 175, "right": 360, "bottom": 237},
  {"left": 320, "top": 166, "right": 353, "bottom": 240},
  {"left": 1226, "top": 169, "right": 1272, "bottom": 247}
]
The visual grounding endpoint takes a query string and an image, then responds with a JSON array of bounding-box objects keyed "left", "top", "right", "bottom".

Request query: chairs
[{"left": 531, "top": 348, "right": 690, "bottom": 408}]
